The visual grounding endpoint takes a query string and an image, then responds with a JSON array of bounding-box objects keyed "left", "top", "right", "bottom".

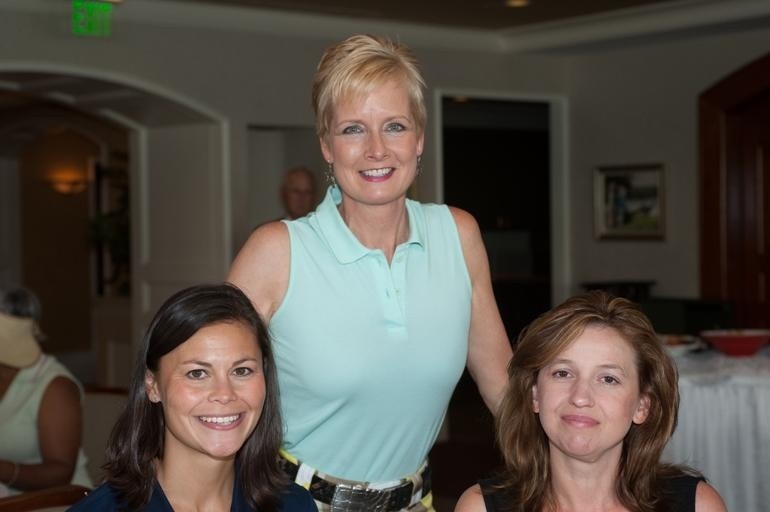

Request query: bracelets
[{"left": 8, "top": 462, "right": 21, "bottom": 486}]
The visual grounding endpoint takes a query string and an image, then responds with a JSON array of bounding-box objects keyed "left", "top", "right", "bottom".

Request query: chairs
[{"left": 1, "top": 482, "right": 90, "bottom": 512}]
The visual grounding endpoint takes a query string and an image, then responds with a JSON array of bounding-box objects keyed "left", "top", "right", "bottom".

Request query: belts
[{"left": 277, "top": 453, "right": 432, "bottom": 512}]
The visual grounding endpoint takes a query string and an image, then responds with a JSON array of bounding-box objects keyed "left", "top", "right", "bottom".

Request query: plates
[{"left": 652, "top": 334, "right": 700, "bottom": 359}]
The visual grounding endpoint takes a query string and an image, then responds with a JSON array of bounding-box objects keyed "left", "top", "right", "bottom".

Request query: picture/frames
[{"left": 590, "top": 160, "right": 669, "bottom": 245}]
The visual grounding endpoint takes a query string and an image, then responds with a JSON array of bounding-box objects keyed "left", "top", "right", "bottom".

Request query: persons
[
  {"left": 0, "top": 285, "right": 93, "bottom": 512},
  {"left": 225, "top": 31, "right": 514, "bottom": 512},
  {"left": 452, "top": 285, "right": 728, "bottom": 512},
  {"left": 64, "top": 283, "right": 320, "bottom": 512},
  {"left": 279, "top": 168, "right": 317, "bottom": 218}
]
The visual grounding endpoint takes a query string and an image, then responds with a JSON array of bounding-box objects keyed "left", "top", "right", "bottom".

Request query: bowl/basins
[{"left": 701, "top": 329, "right": 770, "bottom": 358}]
